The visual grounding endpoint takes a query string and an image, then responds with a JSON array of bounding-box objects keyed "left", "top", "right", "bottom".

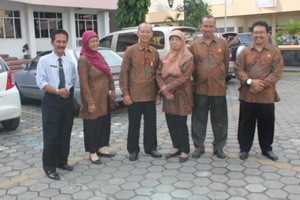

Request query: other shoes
[
  {"left": 128, "top": 152, "right": 137, "bottom": 161},
  {"left": 170, "top": 147, "right": 179, "bottom": 156},
  {"left": 192, "top": 147, "right": 203, "bottom": 158},
  {"left": 89, "top": 153, "right": 100, "bottom": 164},
  {"left": 97, "top": 150, "right": 114, "bottom": 157},
  {"left": 150, "top": 150, "right": 161, "bottom": 158},
  {"left": 238, "top": 151, "right": 247, "bottom": 160},
  {"left": 213, "top": 147, "right": 224, "bottom": 158},
  {"left": 261, "top": 150, "right": 277, "bottom": 161},
  {"left": 178, "top": 152, "right": 188, "bottom": 162}
]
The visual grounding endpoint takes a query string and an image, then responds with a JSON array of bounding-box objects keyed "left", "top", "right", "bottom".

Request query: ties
[{"left": 57, "top": 57, "right": 64, "bottom": 89}]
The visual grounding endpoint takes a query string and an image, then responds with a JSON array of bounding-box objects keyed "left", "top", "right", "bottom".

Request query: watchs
[{"left": 244, "top": 78, "right": 252, "bottom": 87}]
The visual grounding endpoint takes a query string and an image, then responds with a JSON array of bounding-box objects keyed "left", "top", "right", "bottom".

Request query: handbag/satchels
[{"left": 108, "top": 93, "right": 119, "bottom": 112}]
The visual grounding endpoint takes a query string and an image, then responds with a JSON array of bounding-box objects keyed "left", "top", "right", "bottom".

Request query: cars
[
  {"left": 0, "top": 56, "right": 22, "bottom": 131},
  {"left": 13, "top": 47, "right": 124, "bottom": 116}
]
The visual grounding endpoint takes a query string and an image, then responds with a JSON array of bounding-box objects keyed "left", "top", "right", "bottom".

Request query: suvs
[
  {"left": 237, "top": 32, "right": 281, "bottom": 56},
  {"left": 197, "top": 32, "right": 246, "bottom": 83}
]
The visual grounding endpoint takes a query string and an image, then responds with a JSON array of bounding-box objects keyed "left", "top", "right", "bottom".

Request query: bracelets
[{"left": 55, "top": 88, "right": 59, "bottom": 95}]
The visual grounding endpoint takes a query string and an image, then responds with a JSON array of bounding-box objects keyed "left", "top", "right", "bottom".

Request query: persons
[
  {"left": 77, "top": 30, "right": 119, "bottom": 164},
  {"left": 36, "top": 29, "right": 76, "bottom": 179},
  {"left": 188, "top": 15, "right": 230, "bottom": 160},
  {"left": 155, "top": 29, "right": 195, "bottom": 163},
  {"left": 119, "top": 22, "right": 163, "bottom": 161},
  {"left": 228, "top": 21, "right": 284, "bottom": 161}
]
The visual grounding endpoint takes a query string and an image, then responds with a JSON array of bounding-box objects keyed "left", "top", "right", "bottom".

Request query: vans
[{"left": 98, "top": 26, "right": 198, "bottom": 74}]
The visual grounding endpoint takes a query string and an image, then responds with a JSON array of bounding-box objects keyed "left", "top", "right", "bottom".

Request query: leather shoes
[
  {"left": 43, "top": 168, "right": 59, "bottom": 179},
  {"left": 56, "top": 163, "right": 72, "bottom": 170}
]
